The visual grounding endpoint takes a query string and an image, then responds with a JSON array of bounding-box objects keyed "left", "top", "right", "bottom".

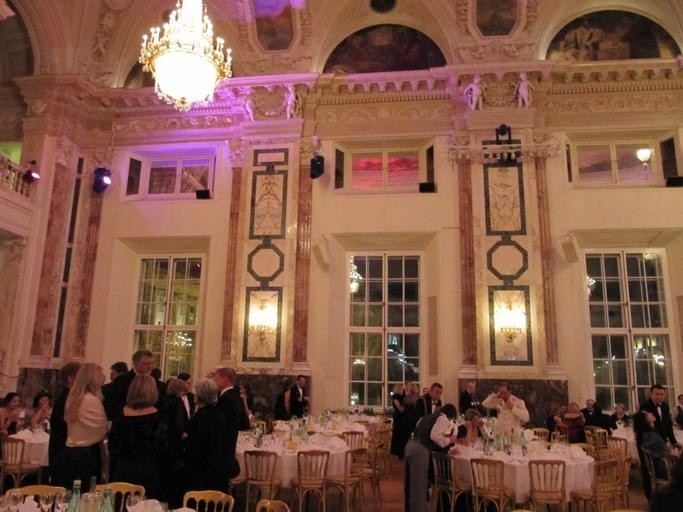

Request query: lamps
[
  {"left": 93, "top": 168, "right": 113, "bottom": 194},
  {"left": 138, "top": 0, "right": 233, "bottom": 113},
  {"left": 248, "top": 296, "right": 277, "bottom": 342},
  {"left": 634, "top": 148, "right": 651, "bottom": 166},
  {"left": 24, "top": 169, "right": 40, "bottom": 184},
  {"left": 493, "top": 297, "right": 529, "bottom": 343}
]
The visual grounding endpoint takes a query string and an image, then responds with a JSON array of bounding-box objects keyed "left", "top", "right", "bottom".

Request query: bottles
[{"left": 66, "top": 475, "right": 112, "bottom": 511}]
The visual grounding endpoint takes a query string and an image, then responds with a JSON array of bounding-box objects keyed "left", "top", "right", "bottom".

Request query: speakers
[
  {"left": 310, "top": 156, "right": 324, "bottom": 178},
  {"left": 419, "top": 183, "right": 434, "bottom": 192},
  {"left": 196, "top": 190, "right": 210, "bottom": 199},
  {"left": 667, "top": 177, "right": 683, "bottom": 186}
]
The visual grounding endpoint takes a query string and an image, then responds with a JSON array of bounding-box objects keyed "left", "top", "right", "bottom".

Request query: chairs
[
  {"left": 5, "top": 481, "right": 291, "bottom": 512},
  {"left": 427, "top": 422, "right": 683, "bottom": 512},
  {"left": 229, "top": 408, "right": 394, "bottom": 512},
  {"left": 0, "top": 435, "right": 42, "bottom": 492}
]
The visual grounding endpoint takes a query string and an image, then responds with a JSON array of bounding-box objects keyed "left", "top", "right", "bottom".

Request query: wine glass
[
  {"left": 17, "top": 423, "right": 48, "bottom": 441},
  {"left": 458, "top": 433, "right": 581, "bottom": 461},
  {"left": 615, "top": 420, "right": 634, "bottom": 436},
  {"left": 242, "top": 410, "right": 380, "bottom": 454},
  {"left": 1, "top": 490, "right": 174, "bottom": 512},
  {"left": 672, "top": 423, "right": 680, "bottom": 439}
]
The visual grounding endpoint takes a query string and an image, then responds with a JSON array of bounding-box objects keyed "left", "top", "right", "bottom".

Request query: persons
[
  {"left": 632, "top": 384, "right": 682, "bottom": 512},
  {"left": 48, "top": 346, "right": 254, "bottom": 511},
  {"left": 1, "top": 390, "right": 52, "bottom": 495},
  {"left": 274, "top": 375, "right": 311, "bottom": 423},
  {"left": 545, "top": 398, "right": 631, "bottom": 446},
  {"left": 390, "top": 380, "right": 538, "bottom": 512}
]
